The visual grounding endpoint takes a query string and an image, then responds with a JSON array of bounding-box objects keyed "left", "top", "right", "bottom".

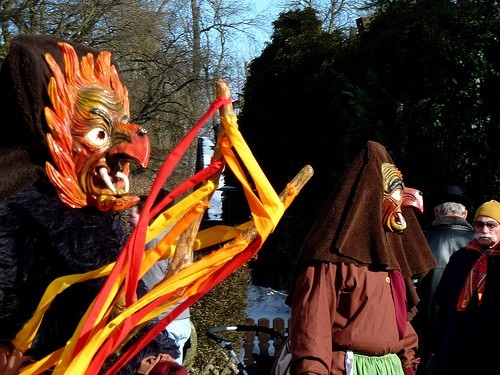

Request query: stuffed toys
[{"left": 0, "top": 32, "right": 188, "bottom": 375}]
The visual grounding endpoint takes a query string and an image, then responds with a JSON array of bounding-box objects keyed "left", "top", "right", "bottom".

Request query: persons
[
  {"left": 126, "top": 189, "right": 192, "bottom": 366},
  {"left": 285, "top": 141, "right": 438, "bottom": 375},
  {"left": 412, "top": 186, "right": 500, "bottom": 375}
]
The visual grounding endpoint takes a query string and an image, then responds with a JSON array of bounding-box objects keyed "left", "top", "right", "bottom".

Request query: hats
[
  {"left": 431, "top": 186, "right": 468, "bottom": 209},
  {"left": 473, "top": 200, "right": 500, "bottom": 224}
]
